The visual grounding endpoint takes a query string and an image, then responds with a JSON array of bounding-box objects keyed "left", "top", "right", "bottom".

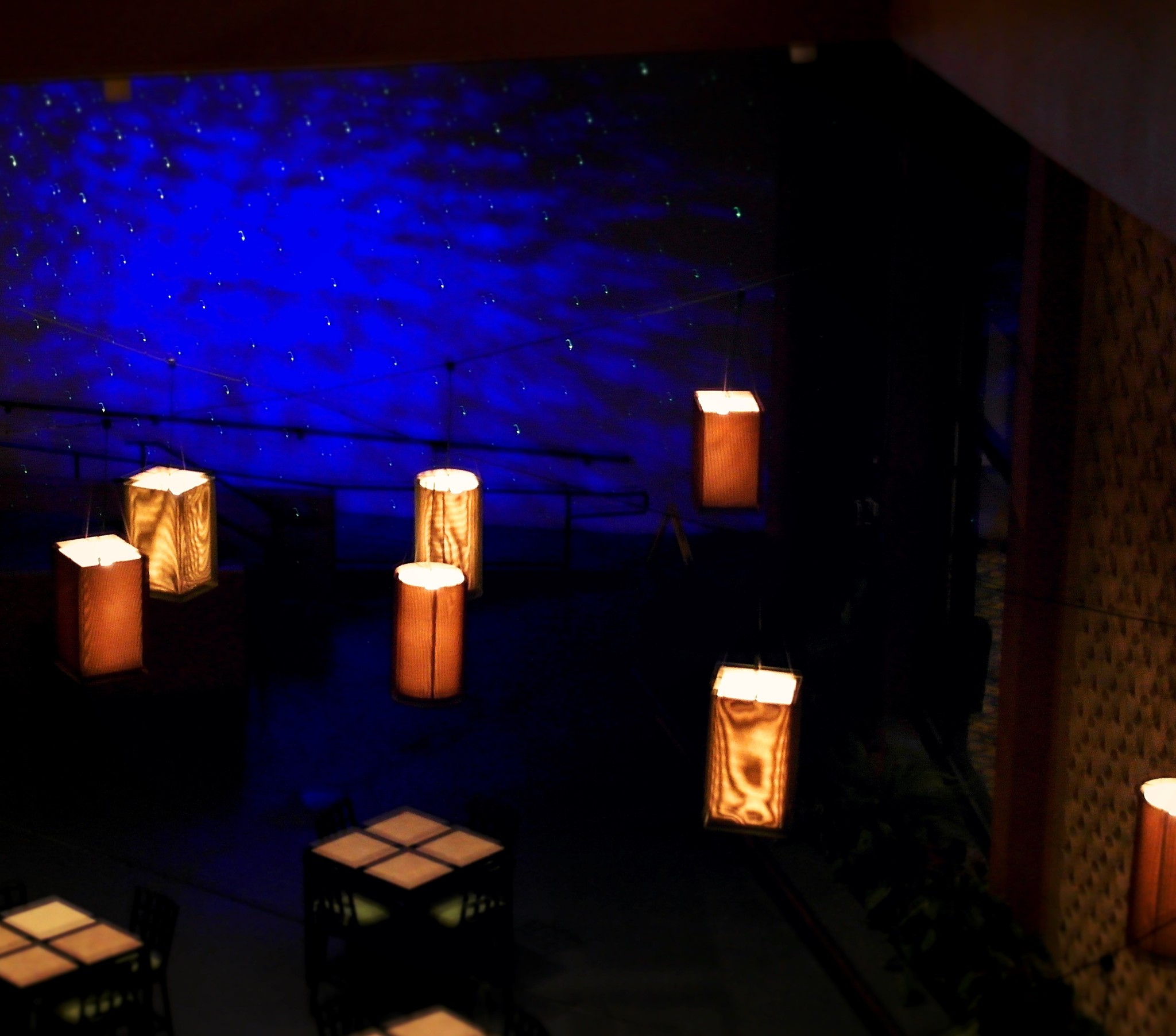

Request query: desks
[
  {"left": 311, "top": 804, "right": 505, "bottom": 1014},
  {"left": 0, "top": 896, "right": 142, "bottom": 1036}
]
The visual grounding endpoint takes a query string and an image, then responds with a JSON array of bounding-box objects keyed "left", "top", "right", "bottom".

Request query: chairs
[
  {"left": 128, "top": 884, "right": 180, "bottom": 1036},
  {"left": 0, "top": 882, "right": 26, "bottom": 911},
  {"left": 310, "top": 796, "right": 510, "bottom": 1018}
]
